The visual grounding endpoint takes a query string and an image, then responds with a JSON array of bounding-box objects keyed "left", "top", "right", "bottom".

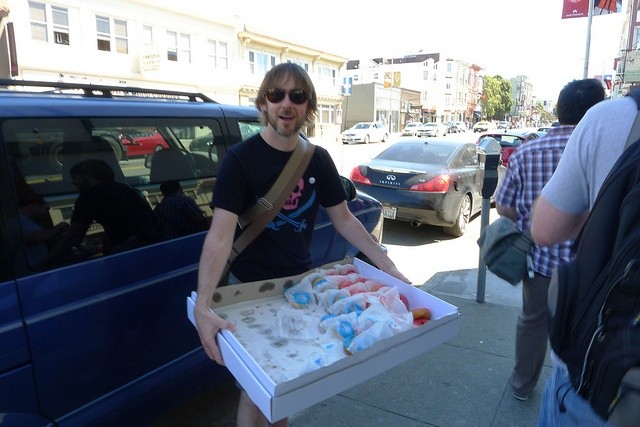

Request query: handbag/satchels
[{"left": 477, "top": 215, "right": 532, "bottom": 285}]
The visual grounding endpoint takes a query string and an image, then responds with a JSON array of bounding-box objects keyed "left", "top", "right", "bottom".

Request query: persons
[
  {"left": 490, "top": 78, "right": 609, "bottom": 402},
  {"left": 194, "top": 61, "right": 411, "bottom": 423},
  {"left": 154, "top": 180, "right": 215, "bottom": 235},
  {"left": 529, "top": 83, "right": 639, "bottom": 427},
  {"left": 21, "top": 179, "right": 64, "bottom": 271},
  {"left": 69, "top": 161, "right": 157, "bottom": 259}
]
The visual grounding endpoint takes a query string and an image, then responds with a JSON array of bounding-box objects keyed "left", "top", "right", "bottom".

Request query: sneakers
[{"left": 513, "top": 393, "right": 528, "bottom": 401}]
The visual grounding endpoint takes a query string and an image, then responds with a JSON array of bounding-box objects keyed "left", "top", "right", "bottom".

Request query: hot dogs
[{"left": 307, "top": 264, "right": 432, "bottom": 354}]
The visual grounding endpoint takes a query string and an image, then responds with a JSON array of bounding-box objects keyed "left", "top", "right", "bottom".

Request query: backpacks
[{"left": 546, "top": 87, "right": 639, "bottom": 425}]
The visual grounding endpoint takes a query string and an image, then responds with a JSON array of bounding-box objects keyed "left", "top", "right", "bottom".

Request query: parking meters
[{"left": 475, "top": 137, "right": 504, "bottom": 303}]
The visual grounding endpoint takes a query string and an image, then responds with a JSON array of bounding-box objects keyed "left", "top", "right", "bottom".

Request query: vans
[{"left": 0, "top": 75, "right": 390, "bottom": 426}]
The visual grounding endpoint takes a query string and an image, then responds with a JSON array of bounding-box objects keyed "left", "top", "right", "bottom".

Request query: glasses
[{"left": 264, "top": 87, "right": 311, "bottom": 104}]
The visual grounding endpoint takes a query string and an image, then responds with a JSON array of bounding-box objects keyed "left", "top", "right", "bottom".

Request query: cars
[
  {"left": 473, "top": 120, "right": 493, "bottom": 131},
  {"left": 476, "top": 127, "right": 540, "bottom": 167},
  {"left": 402, "top": 121, "right": 423, "bottom": 136},
  {"left": 536, "top": 126, "right": 555, "bottom": 135},
  {"left": 498, "top": 120, "right": 510, "bottom": 128},
  {"left": 117, "top": 129, "right": 171, "bottom": 158},
  {"left": 342, "top": 121, "right": 388, "bottom": 143},
  {"left": 417, "top": 121, "right": 447, "bottom": 138},
  {"left": 455, "top": 120, "right": 466, "bottom": 132},
  {"left": 351, "top": 137, "right": 506, "bottom": 237},
  {"left": 442, "top": 121, "right": 457, "bottom": 133}
]
